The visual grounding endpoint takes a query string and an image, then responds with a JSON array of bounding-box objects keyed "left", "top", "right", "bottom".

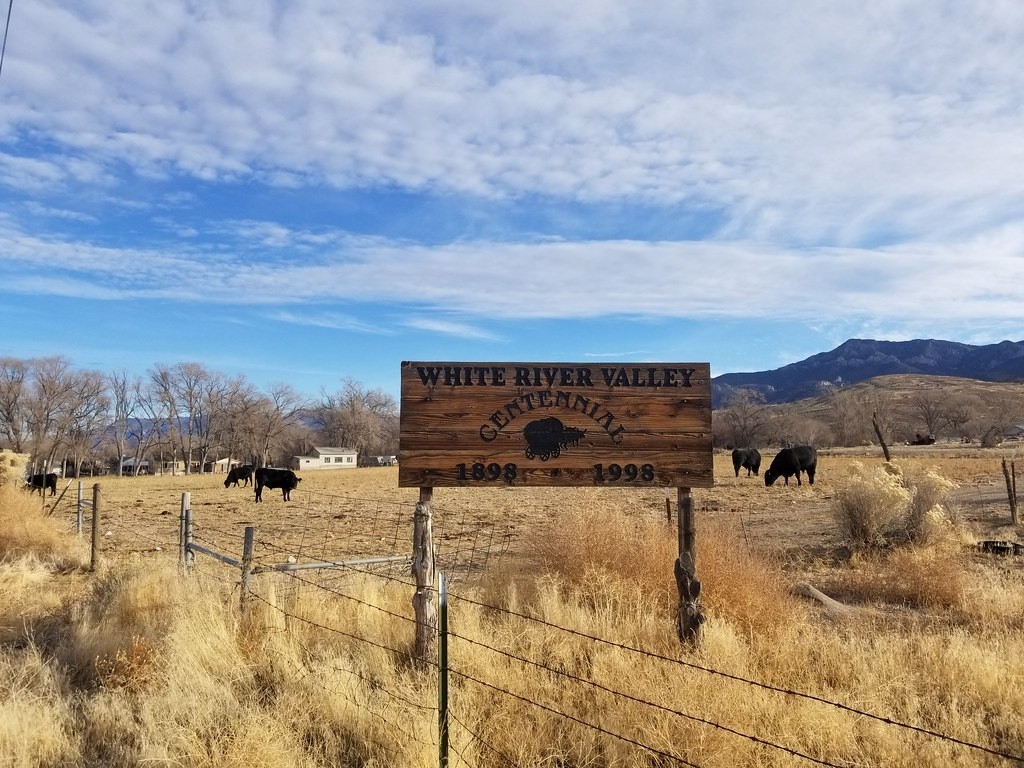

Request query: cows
[
  {"left": 224, "top": 466, "right": 302, "bottom": 503},
  {"left": 732, "top": 446, "right": 817, "bottom": 486},
  {"left": 27, "top": 473, "right": 57, "bottom": 496}
]
